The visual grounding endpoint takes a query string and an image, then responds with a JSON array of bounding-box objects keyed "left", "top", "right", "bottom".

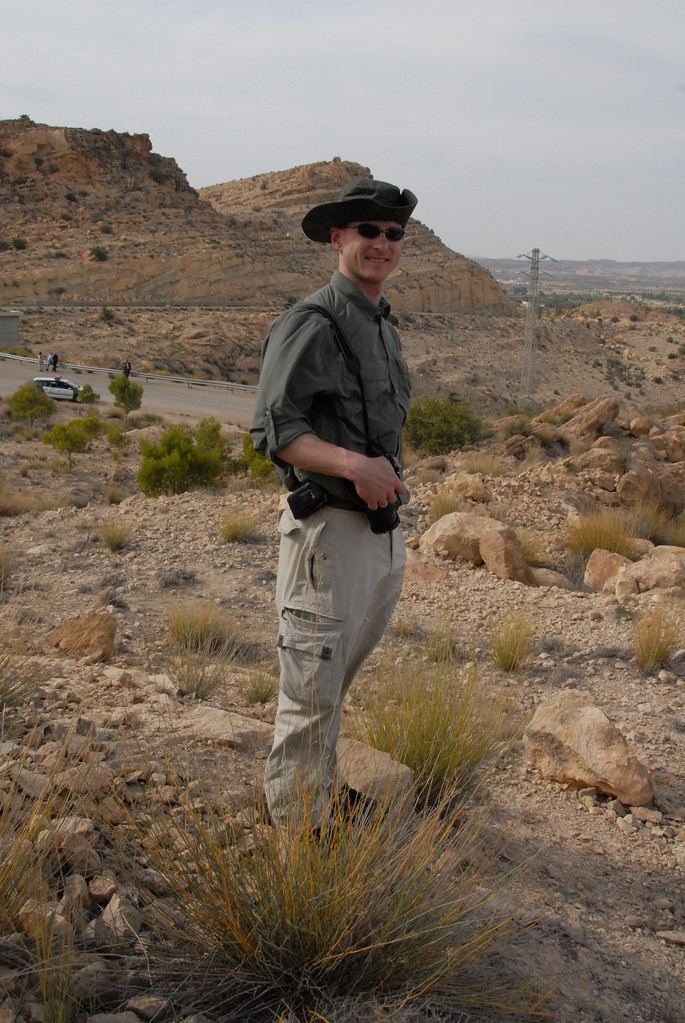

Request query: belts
[{"left": 322, "top": 492, "right": 367, "bottom": 512}]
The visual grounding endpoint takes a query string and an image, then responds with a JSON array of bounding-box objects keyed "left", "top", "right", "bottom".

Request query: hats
[{"left": 301, "top": 179, "right": 417, "bottom": 243}]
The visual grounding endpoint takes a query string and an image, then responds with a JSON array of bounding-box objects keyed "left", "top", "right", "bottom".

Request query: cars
[{"left": 31, "top": 376, "right": 86, "bottom": 404}]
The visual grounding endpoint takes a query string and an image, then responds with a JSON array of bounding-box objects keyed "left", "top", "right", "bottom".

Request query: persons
[
  {"left": 124, "top": 358, "right": 131, "bottom": 379},
  {"left": 252, "top": 180, "right": 417, "bottom": 843},
  {"left": 38, "top": 351, "right": 59, "bottom": 371}
]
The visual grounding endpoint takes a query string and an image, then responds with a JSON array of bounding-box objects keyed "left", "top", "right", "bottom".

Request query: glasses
[{"left": 340, "top": 223, "right": 406, "bottom": 242}]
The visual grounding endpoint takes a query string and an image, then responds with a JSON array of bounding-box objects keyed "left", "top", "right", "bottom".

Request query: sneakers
[{"left": 333, "top": 786, "right": 389, "bottom": 822}]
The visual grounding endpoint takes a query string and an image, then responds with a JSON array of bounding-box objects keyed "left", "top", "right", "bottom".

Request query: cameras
[{"left": 342, "top": 456, "right": 411, "bottom": 535}]
